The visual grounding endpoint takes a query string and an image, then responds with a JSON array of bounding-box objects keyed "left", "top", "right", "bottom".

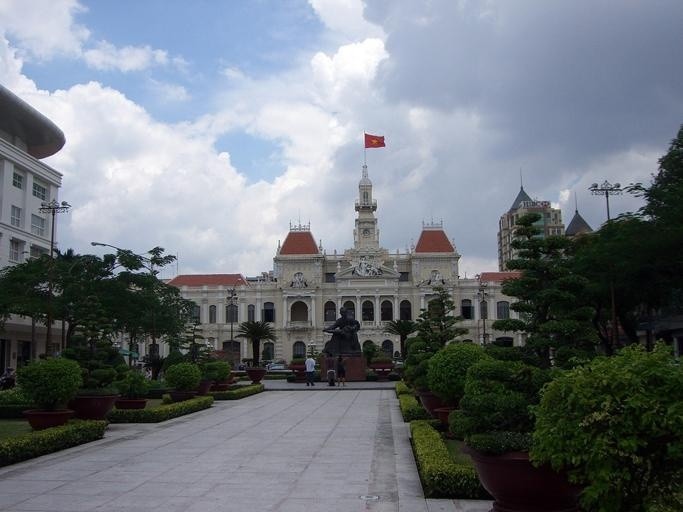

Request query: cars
[
  {"left": 259, "top": 359, "right": 289, "bottom": 371},
  {"left": 393, "top": 357, "right": 404, "bottom": 365},
  {"left": 239, "top": 364, "right": 246, "bottom": 370}
]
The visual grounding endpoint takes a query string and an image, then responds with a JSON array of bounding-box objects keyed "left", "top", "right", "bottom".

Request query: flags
[{"left": 365, "top": 134, "right": 385, "bottom": 148}]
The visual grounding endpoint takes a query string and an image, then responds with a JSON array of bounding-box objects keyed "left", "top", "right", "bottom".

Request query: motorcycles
[{"left": 0, "top": 376, "right": 15, "bottom": 390}]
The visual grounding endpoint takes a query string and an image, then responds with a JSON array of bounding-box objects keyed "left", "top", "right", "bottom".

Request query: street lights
[
  {"left": 91, "top": 241, "right": 120, "bottom": 250},
  {"left": 227, "top": 278, "right": 241, "bottom": 352},
  {"left": 587, "top": 179, "right": 623, "bottom": 346},
  {"left": 475, "top": 274, "right": 487, "bottom": 348},
  {"left": 38, "top": 198, "right": 72, "bottom": 259}
]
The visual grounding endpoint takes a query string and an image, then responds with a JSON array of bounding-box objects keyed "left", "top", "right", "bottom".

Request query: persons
[
  {"left": 336, "top": 354, "right": 347, "bottom": 387},
  {"left": 305, "top": 354, "right": 315, "bottom": 386},
  {"left": 146, "top": 367, "right": 152, "bottom": 380},
  {"left": 321, "top": 307, "right": 361, "bottom": 353},
  {"left": 0, "top": 367, "right": 16, "bottom": 387}
]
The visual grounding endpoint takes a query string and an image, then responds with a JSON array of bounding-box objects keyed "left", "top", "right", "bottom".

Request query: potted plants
[
  {"left": 403, "top": 343, "right": 593, "bottom": 511},
  {"left": 16, "top": 339, "right": 235, "bottom": 430},
  {"left": 235, "top": 319, "right": 279, "bottom": 385}
]
